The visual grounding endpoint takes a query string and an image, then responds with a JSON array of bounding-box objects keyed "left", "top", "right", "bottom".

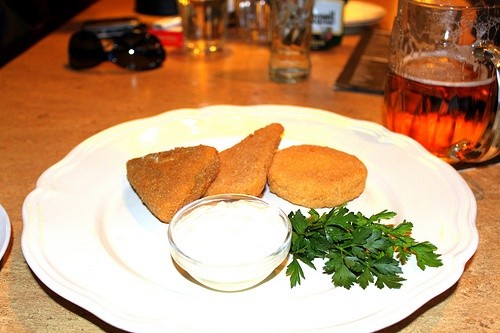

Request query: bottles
[{"left": 282, "top": 0, "right": 345, "bottom": 51}]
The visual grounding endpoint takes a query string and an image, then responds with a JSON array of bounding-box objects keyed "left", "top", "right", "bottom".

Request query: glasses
[{"left": 68, "top": 28, "right": 167, "bottom": 70}]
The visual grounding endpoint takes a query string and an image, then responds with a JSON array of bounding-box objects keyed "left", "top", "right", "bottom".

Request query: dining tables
[{"left": 0, "top": 0, "right": 500, "bottom": 333}]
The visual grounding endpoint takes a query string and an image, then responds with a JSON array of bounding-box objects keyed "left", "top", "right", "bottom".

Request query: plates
[
  {"left": 19, "top": 103, "right": 480, "bottom": 333},
  {"left": 0, "top": 204, "right": 11, "bottom": 260},
  {"left": 340, "top": 1, "right": 386, "bottom": 28}
]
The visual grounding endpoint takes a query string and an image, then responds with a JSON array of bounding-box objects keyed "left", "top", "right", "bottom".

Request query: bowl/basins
[{"left": 167, "top": 192, "right": 292, "bottom": 293}]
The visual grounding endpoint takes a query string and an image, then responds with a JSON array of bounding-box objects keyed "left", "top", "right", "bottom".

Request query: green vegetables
[{"left": 286, "top": 204, "right": 443, "bottom": 289}]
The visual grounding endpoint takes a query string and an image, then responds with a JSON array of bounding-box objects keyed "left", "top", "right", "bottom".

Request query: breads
[
  {"left": 200, "top": 122, "right": 283, "bottom": 198},
  {"left": 126, "top": 144, "right": 220, "bottom": 223},
  {"left": 266, "top": 144, "right": 368, "bottom": 209}
]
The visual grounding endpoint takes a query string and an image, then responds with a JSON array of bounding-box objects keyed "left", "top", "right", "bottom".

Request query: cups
[
  {"left": 178, "top": 0, "right": 227, "bottom": 57},
  {"left": 264, "top": 0, "right": 315, "bottom": 85},
  {"left": 234, "top": 0, "right": 272, "bottom": 46},
  {"left": 381, "top": 0, "right": 500, "bottom": 168}
]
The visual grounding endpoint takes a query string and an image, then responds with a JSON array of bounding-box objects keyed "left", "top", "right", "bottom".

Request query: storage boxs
[{"left": 147, "top": 26, "right": 185, "bottom": 50}]
